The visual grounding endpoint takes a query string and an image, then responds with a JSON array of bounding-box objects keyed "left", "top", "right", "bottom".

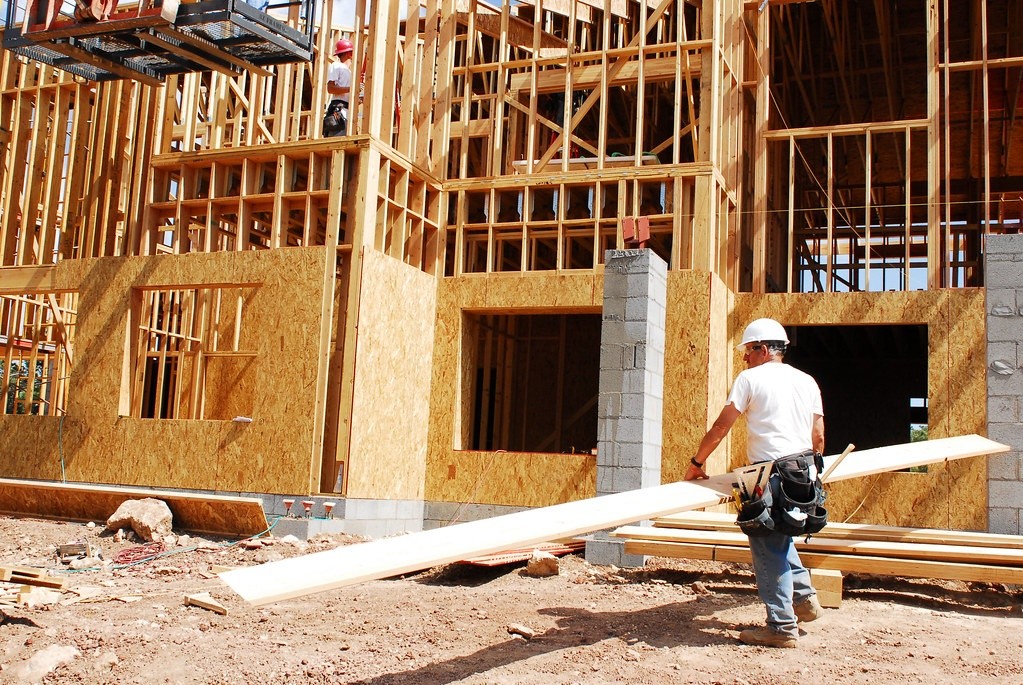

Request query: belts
[{"left": 800, "top": 456, "right": 816, "bottom": 467}]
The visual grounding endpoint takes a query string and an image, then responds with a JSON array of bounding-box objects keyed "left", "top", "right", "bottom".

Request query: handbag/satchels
[{"left": 323, "top": 103, "right": 346, "bottom": 135}]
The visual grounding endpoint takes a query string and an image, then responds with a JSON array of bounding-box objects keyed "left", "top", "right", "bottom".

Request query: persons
[
  {"left": 323, "top": 40, "right": 402, "bottom": 136},
  {"left": 685, "top": 318, "right": 824, "bottom": 647}
]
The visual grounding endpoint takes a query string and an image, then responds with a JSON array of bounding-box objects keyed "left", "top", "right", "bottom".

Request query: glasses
[{"left": 743, "top": 344, "right": 761, "bottom": 355}]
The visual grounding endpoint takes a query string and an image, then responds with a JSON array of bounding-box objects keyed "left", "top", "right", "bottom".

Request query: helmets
[
  {"left": 333, "top": 39, "right": 353, "bottom": 56},
  {"left": 736, "top": 318, "right": 790, "bottom": 350}
]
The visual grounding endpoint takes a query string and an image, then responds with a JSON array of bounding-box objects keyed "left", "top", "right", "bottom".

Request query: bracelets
[{"left": 691, "top": 457, "right": 703, "bottom": 467}]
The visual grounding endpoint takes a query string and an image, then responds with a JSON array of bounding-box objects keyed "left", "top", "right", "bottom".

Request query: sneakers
[
  {"left": 792, "top": 594, "right": 824, "bottom": 623},
  {"left": 739, "top": 626, "right": 796, "bottom": 648}
]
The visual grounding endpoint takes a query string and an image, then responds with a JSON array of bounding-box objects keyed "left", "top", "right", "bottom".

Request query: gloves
[{"left": 360, "top": 83, "right": 364, "bottom": 90}]
